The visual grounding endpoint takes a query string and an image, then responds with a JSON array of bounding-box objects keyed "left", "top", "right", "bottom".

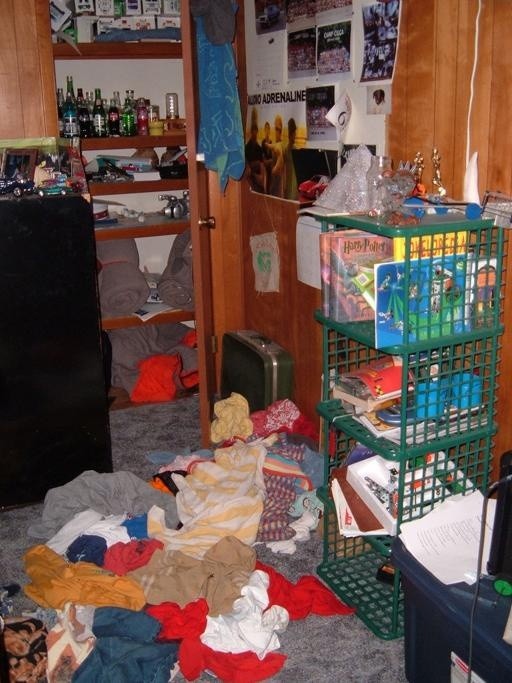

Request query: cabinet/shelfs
[
  {"left": 313, "top": 208, "right": 509, "bottom": 639},
  {"left": 0, "top": 0, "right": 245, "bottom": 449}
]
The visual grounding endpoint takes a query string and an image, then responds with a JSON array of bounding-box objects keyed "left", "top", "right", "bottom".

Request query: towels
[{"left": 195, "top": 16, "right": 245, "bottom": 196}]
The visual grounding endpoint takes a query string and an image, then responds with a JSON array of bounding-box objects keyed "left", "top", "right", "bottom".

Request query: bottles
[
  {"left": 366, "top": 154, "right": 395, "bottom": 211},
  {"left": 57, "top": 76, "right": 148, "bottom": 138}
]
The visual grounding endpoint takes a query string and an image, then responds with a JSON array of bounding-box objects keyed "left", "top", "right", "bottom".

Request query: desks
[
  {"left": 391, "top": 526, "right": 512, "bottom": 683},
  {"left": 0, "top": 195, "right": 113, "bottom": 510}
]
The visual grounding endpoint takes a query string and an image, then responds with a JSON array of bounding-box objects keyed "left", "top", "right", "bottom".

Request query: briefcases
[{"left": 220, "top": 329, "right": 294, "bottom": 416}]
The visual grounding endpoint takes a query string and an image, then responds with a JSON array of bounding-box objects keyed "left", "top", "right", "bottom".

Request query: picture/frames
[{"left": 1, "top": 148, "right": 38, "bottom": 186}]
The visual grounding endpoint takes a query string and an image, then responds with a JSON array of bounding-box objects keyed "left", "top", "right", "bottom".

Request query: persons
[
  {"left": 261, "top": 122, "right": 272, "bottom": 158},
  {"left": 273, "top": 117, "right": 299, "bottom": 202},
  {"left": 271, "top": 114, "right": 284, "bottom": 153},
  {"left": 244, "top": 106, "right": 265, "bottom": 193}
]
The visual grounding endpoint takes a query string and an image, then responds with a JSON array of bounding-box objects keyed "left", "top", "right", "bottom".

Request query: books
[
  {"left": 317, "top": 227, "right": 498, "bottom": 349},
  {"left": 332, "top": 348, "right": 489, "bottom": 447},
  {"left": 329, "top": 441, "right": 478, "bottom": 540}
]
